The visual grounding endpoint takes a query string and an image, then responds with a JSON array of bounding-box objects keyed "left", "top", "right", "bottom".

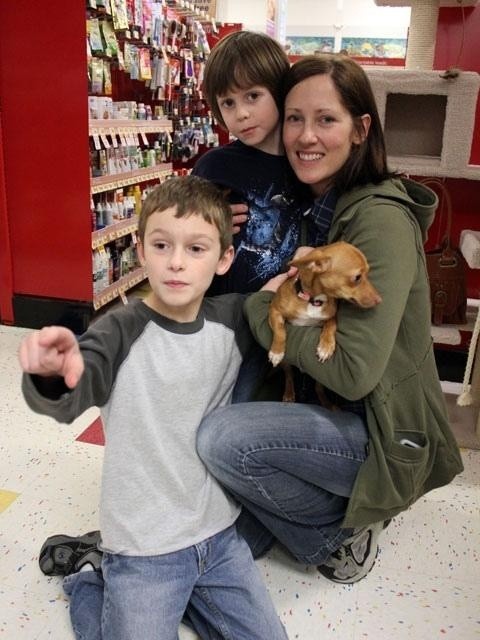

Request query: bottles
[
  {"left": 89, "top": 186, "right": 142, "bottom": 232},
  {"left": 137, "top": 102, "right": 146, "bottom": 120},
  {"left": 92, "top": 245, "right": 130, "bottom": 295},
  {"left": 153, "top": 140, "right": 161, "bottom": 163}
]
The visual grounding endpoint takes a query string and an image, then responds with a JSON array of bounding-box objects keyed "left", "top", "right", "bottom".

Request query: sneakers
[
  {"left": 317, "top": 519, "right": 391, "bottom": 583},
  {"left": 39, "top": 531, "right": 103, "bottom": 579}
]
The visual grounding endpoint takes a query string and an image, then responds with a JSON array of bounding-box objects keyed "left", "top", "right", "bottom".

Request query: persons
[
  {"left": 191, "top": 29, "right": 323, "bottom": 407},
  {"left": 16, "top": 177, "right": 288, "bottom": 640},
  {"left": 198, "top": 51, "right": 465, "bottom": 583}
]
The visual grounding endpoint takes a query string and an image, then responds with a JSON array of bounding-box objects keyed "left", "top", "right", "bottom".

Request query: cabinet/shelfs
[{"left": 0, "top": 0, "right": 245, "bottom": 336}]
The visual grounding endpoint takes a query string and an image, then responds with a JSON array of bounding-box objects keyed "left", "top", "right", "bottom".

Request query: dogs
[{"left": 266, "top": 240, "right": 383, "bottom": 369}]
